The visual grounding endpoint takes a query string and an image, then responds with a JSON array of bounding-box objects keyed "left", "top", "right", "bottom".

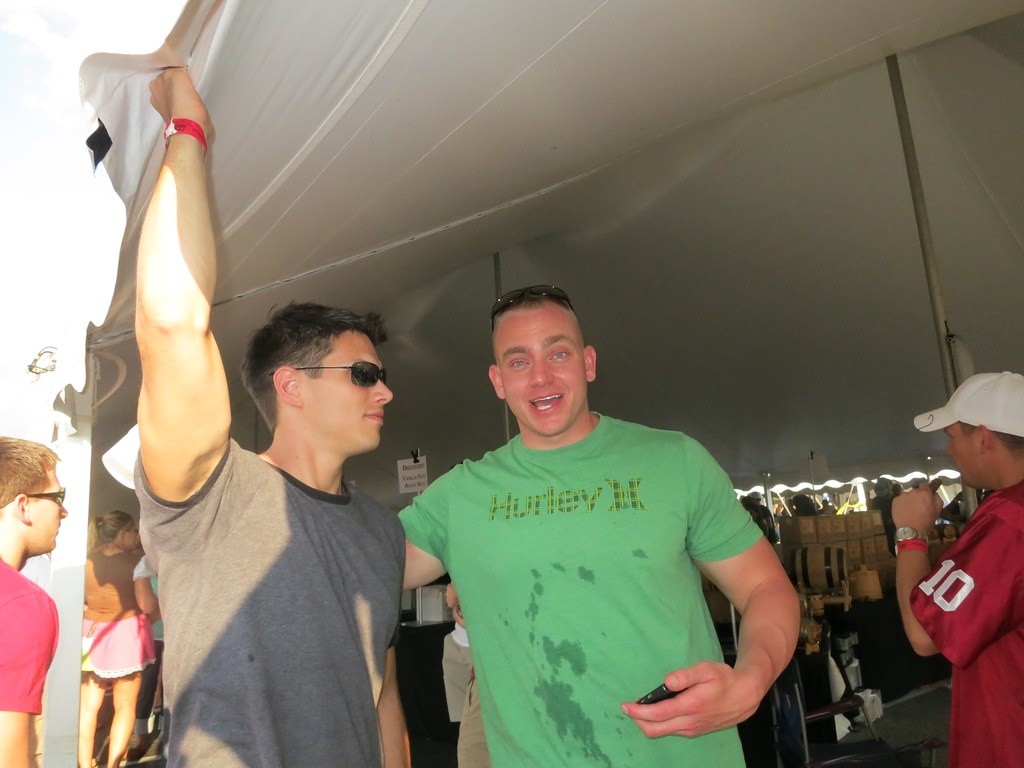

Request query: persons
[
  {"left": 79, "top": 509, "right": 158, "bottom": 768},
  {"left": 126, "top": 556, "right": 168, "bottom": 757},
  {"left": 444, "top": 583, "right": 493, "bottom": 768},
  {"left": 134, "top": 61, "right": 412, "bottom": 768},
  {"left": 0, "top": 436, "right": 69, "bottom": 768},
  {"left": 891, "top": 371, "right": 1024, "bottom": 768},
  {"left": 748, "top": 491, "right": 777, "bottom": 549},
  {"left": 884, "top": 485, "right": 904, "bottom": 555},
  {"left": 819, "top": 499, "right": 835, "bottom": 514},
  {"left": 393, "top": 286, "right": 800, "bottom": 767},
  {"left": 743, "top": 496, "right": 769, "bottom": 537}
]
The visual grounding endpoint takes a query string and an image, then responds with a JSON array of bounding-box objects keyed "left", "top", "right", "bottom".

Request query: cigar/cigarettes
[{"left": 929, "top": 479, "right": 942, "bottom": 494}]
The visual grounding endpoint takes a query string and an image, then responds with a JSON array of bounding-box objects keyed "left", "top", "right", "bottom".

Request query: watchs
[{"left": 894, "top": 526, "right": 930, "bottom": 545}]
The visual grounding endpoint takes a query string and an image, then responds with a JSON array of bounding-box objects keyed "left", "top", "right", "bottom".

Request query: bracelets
[
  {"left": 894, "top": 539, "right": 929, "bottom": 554},
  {"left": 164, "top": 117, "right": 210, "bottom": 161}
]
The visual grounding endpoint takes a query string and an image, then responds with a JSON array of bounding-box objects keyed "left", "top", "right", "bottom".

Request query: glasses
[
  {"left": 491, "top": 285, "right": 574, "bottom": 330},
  {"left": 26, "top": 487, "right": 66, "bottom": 505},
  {"left": 269, "top": 361, "right": 386, "bottom": 389}
]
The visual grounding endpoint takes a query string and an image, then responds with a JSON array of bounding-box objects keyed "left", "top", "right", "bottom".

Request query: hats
[{"left": 914, "top": 372, "right": 1024, "bottom": 436}]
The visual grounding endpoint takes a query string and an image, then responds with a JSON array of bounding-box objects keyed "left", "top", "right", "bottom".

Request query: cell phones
[{"left": 636, "top": 684, "right": 687, "bottom": 705}]
